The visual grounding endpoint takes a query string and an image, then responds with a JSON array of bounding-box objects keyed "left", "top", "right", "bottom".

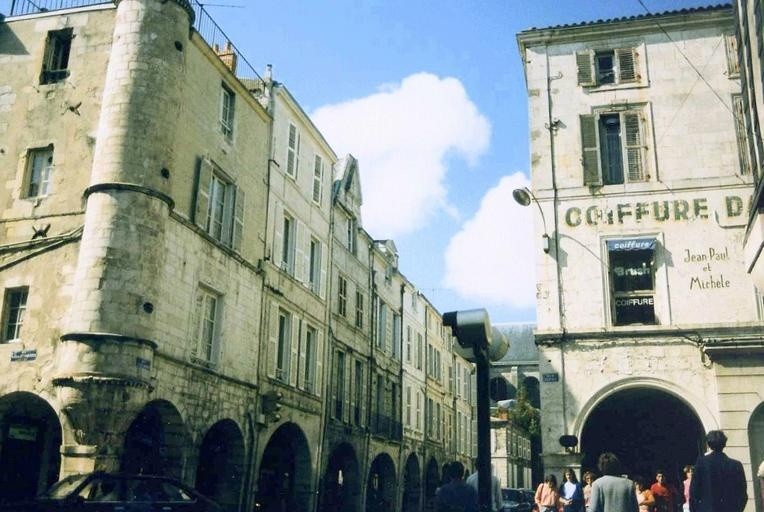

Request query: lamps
[{"left": 512, "top": 186, "right": 550, "bottom": 254}]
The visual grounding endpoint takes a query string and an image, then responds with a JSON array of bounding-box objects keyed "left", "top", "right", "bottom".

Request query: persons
[
  {"left": 689, "top": 430, "right": 748, "bottom": 512},
  {"left": 95, "top": 479, "right": 116, "bottom": 501},
  {"left": 534, "top": 452, "right": 694, "bottom": 512},
  {"left": 756, "top": 460, "right": 764, "bottom": 512},
  {"left": 466, "top": 455, "right": 504, "bottom": 512},
  {"left": 437, "top": 461, "right": 479, "bottom": 512}
]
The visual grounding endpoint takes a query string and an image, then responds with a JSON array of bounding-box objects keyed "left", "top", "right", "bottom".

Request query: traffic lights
[
  {"left": 442, "top": 307, "right": 492, "bottom": 348},
  {"left": 266, "top": 391, "right": 283, "bottom": 422}
]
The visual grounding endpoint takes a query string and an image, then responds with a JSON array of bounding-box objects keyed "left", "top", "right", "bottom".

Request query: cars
[
  {"left": 499, "top": 486, "right": 535, "bottom": 510},
  {"left": 0, "top": 473, "right": 223, "bottom": 512}
]
